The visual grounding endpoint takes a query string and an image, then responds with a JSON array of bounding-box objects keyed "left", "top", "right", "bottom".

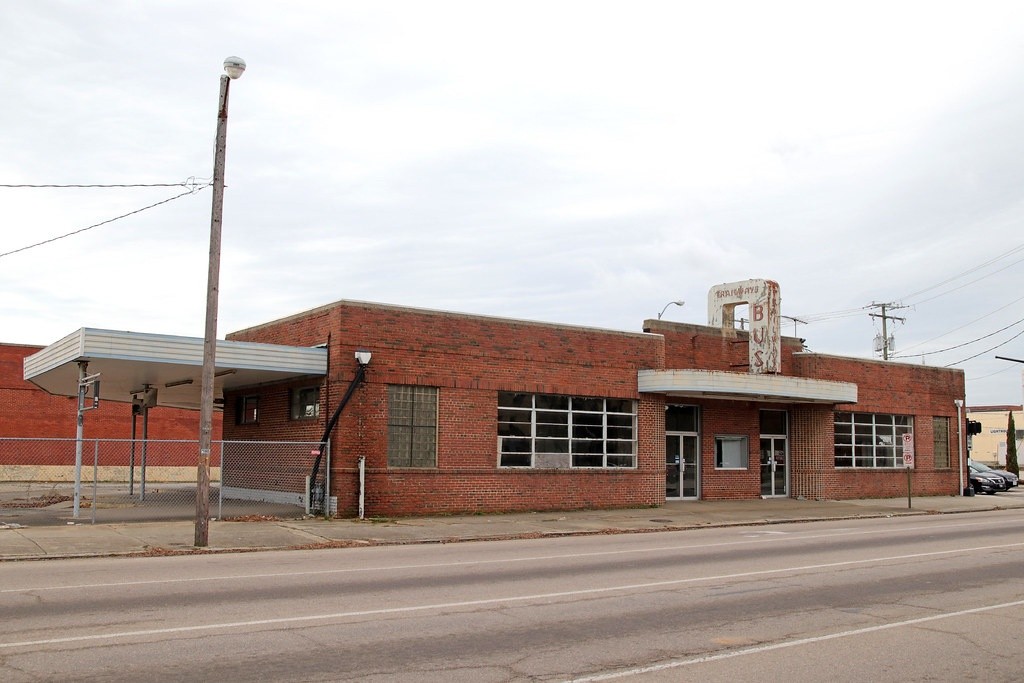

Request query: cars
[
  {"left": 969, "top": 464, "right": 1005, "bottom": 495},
  {"left": 968, "top": 458, "right": 1019, "bottom": 492}
]
